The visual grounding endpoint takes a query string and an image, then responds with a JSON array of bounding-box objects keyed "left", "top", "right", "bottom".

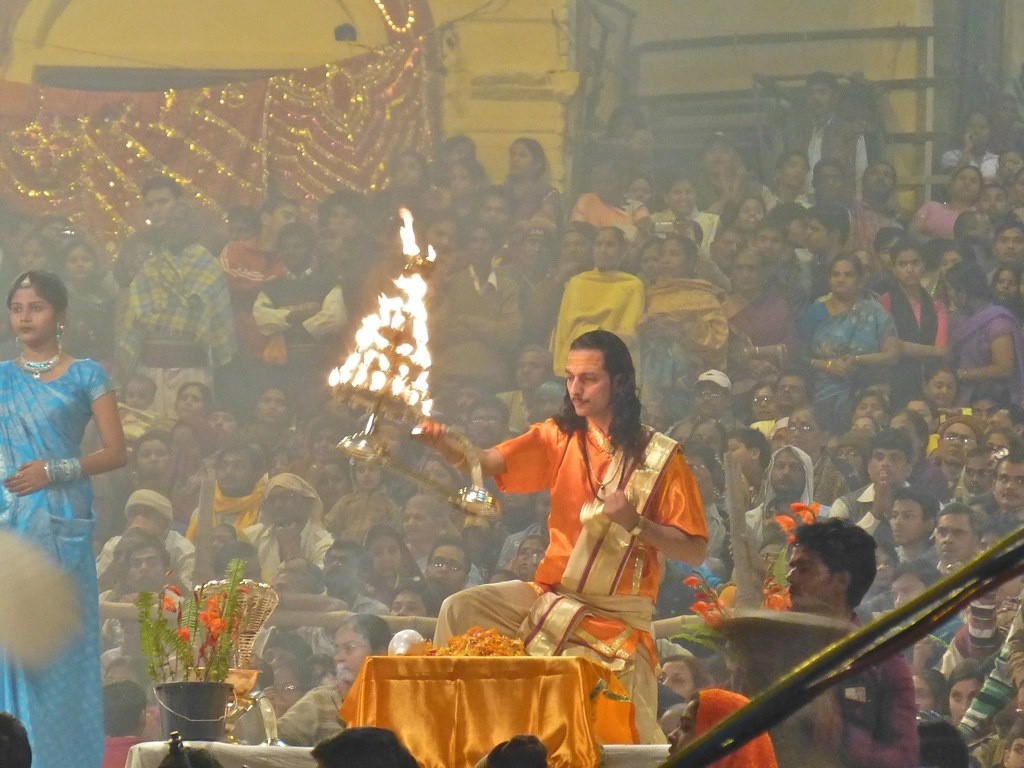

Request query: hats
[
  {"left": 695, "top": 369, "right": 732, "bottom": 392},
  {"left": 125, "top": 489, "right": 173, "bottom": 522}
]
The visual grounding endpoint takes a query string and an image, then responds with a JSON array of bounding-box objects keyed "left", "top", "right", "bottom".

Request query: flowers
[{"left": 132, "top": 556, "right": 249, "bottom": 685}]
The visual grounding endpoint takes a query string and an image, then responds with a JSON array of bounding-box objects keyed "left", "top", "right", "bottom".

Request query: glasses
[
  {"left": 834, "top": 450, "right": 856, "bottom": 457},
  {"left": 789, "top": 423, "right": 813, "bottom": 432},
  {"left": 431, "top": 559, "right": 463, "bottom": 572},
  {"left": 470, "top": 416, "right": 500, "bottom": 429},
  {"left": 323, "top": 558, "right": 351, "bottom": 567},
  {"left": 697, "top": 390, "right": 723, "bottom": 399},
  {"left": 941, "top": 435, "right": 971, "bottom": 445},
  {"left": 752, "top": 395, "right": 771, "bottom": 405}
]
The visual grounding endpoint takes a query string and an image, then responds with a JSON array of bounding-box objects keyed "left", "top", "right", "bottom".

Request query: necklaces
[
  {"left": 582, "top": 430, "right": 625, "bottom": 503},
  {"left": 584, "top": 434, "right": 624, "bottom": 490},
  {"left": 382, "top": 574, "right": 400, "bottom": 593},
  {"left": 19, "top": 351, "right": 62, "bottom": 379}
]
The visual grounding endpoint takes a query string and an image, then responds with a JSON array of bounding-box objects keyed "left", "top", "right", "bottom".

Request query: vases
[{"left": 152, "top": 682, "right": 234, "bottom": 742}]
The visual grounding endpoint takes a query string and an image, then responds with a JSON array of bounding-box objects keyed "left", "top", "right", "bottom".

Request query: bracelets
[
  {"left": 961, "top": 367, "right": 969, "bottom": 383},
  {"left": 854, "top": 354, "right": 861, "bottom": 367},
  {"left": 447, "top": 453, "right": 466, "bottom": 467},
  {"left": 810, "top": 358, "right": 816, "bottom": 364},
  {"left": 759, "top": 566, "right": 770, "bottom": 578},
  {"left": 841, "top": 353, "right": 850, "bottom": 363},
  {"left": 823, "top": 360, "right": 833, "bottom": 374},
  {"left": 628, "top": 515, "right": 645, "bottom": 537},
  {"left": 43, "top": 457, "right": 81, "bottom": 484}
]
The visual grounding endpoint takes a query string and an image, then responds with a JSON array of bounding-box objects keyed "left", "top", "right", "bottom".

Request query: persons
[{"left": 0, "top": 73, "right": 1024, "bottom": 768}]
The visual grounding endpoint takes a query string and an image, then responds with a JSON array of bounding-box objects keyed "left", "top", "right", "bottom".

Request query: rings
[
  {"left": 14, "top": 472, "right": 23, "bottom": 479},
  {"left": 411, "top": 428, "right": 425, "bottom": 436}
]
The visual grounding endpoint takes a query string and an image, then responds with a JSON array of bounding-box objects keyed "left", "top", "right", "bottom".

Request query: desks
[
  {"left": 339, "top": 647, "right": 636, "bottom": 768},
  {"left": 125, "top": 736, "right": 320, "bottom": 767}
]
